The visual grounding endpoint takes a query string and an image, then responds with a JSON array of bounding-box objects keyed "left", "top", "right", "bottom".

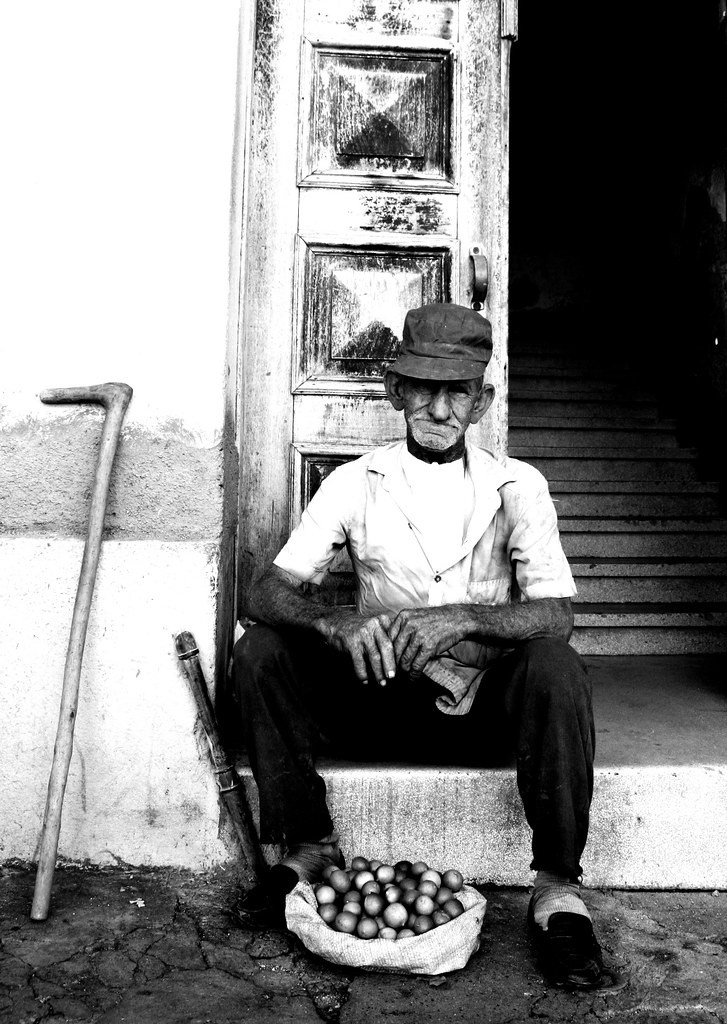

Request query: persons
[{"left": 227, "top": 303, "right": 613, "bottom": 994}]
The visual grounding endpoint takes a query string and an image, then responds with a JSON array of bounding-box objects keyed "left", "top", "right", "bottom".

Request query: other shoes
[
  {"left": 235, "top": 846, "right": 347, "bottom": 916},
  {"left": 527, "top": 894, "right": 603, "bottom": 991}
]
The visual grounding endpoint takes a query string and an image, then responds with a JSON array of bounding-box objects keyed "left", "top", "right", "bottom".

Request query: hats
[{"left": 392, "top": 302, "right": 494, "bottom": 381}]
